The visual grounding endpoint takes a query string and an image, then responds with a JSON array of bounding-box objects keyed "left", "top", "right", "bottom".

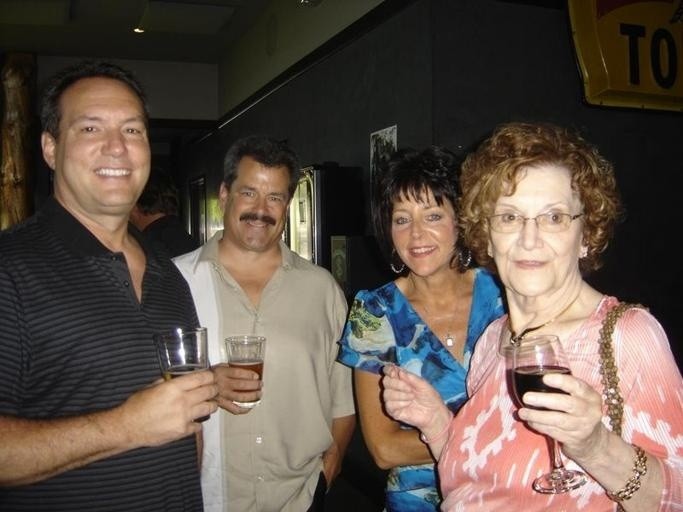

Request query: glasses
[{"left": 486, "top": 211, "right": 586, "bottom": 233}]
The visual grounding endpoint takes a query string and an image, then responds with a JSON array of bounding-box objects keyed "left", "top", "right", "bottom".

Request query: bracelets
[
  {"left": 420, "top": 411, "right": 453, "bottom": 443},
  {"left": 606, "top": 444, "right": 647, "bottom": 503}
]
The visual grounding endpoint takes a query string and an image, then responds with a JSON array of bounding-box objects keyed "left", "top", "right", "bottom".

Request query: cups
[
  {"left": 222, "top": 336, "right": 266, "bottom": 409},
  {"left": 154, "top": 326, "right": 212, "bottom": 421}
]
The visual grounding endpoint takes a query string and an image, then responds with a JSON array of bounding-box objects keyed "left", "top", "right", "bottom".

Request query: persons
[
  {"left": 168, "top": 134, "right": 357, "bottom": 512},
  {"left": 335, "top": 145, "right": 511, "bottom": 512},
  {"left": 0, "top": 61, "right": 219, "bottom": 512},
  {"left": 382, "top": 122, "right": 683, "bottom": 512},
  {"left": 130, "top": 163, "right": 200, "bottom": 260}
]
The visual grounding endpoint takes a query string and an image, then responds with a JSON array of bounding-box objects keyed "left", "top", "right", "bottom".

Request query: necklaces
[
  {"left": 505, "top": 290, "right": 579, "bottom": 347},
  {"left": 412, "top": 275, "right": 464, "bottom": 346}
]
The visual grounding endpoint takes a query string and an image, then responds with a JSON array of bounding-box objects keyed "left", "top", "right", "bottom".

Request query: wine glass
[{"left": 503, "top": 336, "right": 589, "bottom": 495}]
